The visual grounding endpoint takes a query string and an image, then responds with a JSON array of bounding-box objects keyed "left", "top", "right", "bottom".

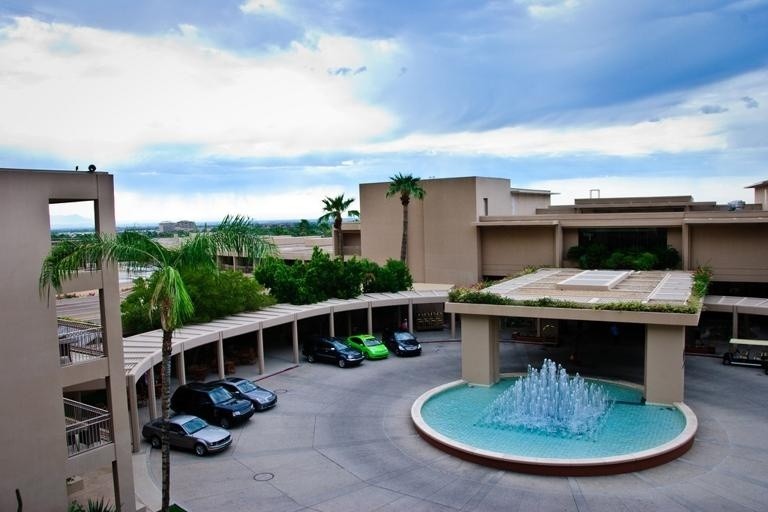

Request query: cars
[
  {"left": 142, "top": 415, "right": 232, "bottom": 456},
  {"left": 207, "top": 378, "right": 277, "bottom": 411}
]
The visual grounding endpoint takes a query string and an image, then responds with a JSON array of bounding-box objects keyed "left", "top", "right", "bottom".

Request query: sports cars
[{"left": 345, "top": 334, "right": 389, "bottom": 359}]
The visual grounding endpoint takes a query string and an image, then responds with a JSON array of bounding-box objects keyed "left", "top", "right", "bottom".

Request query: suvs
[
  {"left": 303, "top": 336, "right": 365, "bottom": 368},
  {"left": 170, "top": 383, "right": 255, "bottom": 429},
  {"left": 382, "top": 327, "right": 422, "bottom": 357}
]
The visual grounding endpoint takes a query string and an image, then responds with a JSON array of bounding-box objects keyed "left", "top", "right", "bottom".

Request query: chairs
[
  {"left": 58, "top": 335, "right": 72, "bottom": 363},
  {"left": 66, "top": 415, "right": 101, "bottom": 451}
]
